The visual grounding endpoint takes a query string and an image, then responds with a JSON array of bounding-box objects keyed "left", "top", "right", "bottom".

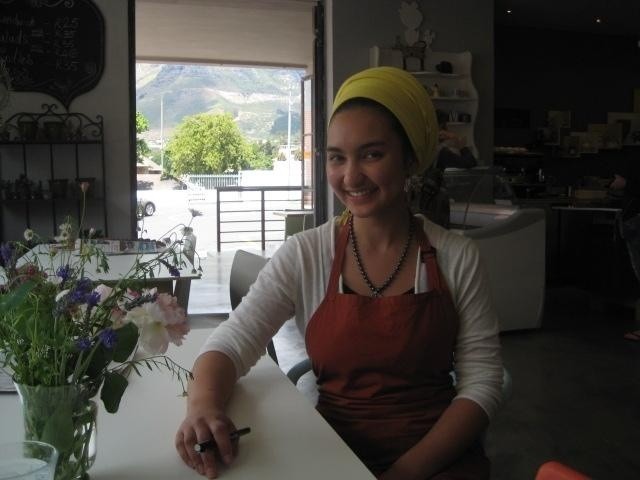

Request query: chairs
[
  {"left": 173, "top": 233, "right": 196, "bottom": 315},
  {"left": 229, "top": 214, "right": 314, "bottom": 386}
]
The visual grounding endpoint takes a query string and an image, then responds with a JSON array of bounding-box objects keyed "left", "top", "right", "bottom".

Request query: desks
[
  {"left": 15, "top": 244, "right": 201, "bottom": 318},
  {"left": 0, "top": 313, "right": 377, "bottom": 480}
]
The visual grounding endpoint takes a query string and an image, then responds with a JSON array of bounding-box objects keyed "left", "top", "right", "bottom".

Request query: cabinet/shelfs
[
  {"left": 368, "top": 46, "right": 480, "bottom": 162},
  {"left": 0, "top": 103, "right": 108, "bottom": 250}
]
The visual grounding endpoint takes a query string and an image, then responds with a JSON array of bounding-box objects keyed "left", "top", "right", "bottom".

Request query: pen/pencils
[{"left": 195, "top": 426, "right": 251, "bottom": 453}]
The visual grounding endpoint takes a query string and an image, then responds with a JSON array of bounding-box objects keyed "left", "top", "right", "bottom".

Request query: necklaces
[{"left": 344, "top": 210, "right": 417, "bottom": 299}]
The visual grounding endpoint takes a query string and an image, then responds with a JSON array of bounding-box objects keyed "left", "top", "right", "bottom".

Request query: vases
[{"left": 12, "top": 371, "right": 98, "bottom": 480}]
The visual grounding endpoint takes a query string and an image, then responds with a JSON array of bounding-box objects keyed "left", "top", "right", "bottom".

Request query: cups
[{"left": 0, "top": 439, "right": 60, "bottom": 479}]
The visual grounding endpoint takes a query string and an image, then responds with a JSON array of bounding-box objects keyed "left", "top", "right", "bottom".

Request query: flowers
[{"left": 0, "top": 182, "right": 203, "bottom": 480}]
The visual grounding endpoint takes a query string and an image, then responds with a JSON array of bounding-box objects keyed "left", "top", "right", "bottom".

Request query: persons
[
  {"left": 418, "top": 108, "right": 474, "bottom": 227},
  {"left": 611, "top": 167, "right": 640, "bottom": 343},
  {"left": 174, "top": 69, "right": 503, "bottom": 479},
  {"left": 180, "top": 226, "right": 197, "bottom": 267}
]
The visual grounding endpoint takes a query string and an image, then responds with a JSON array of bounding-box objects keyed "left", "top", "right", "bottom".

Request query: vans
[{"left": 137, "top": 173, "right": 197, "bottom": 216}]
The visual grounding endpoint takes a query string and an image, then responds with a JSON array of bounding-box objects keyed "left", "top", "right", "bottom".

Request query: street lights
[{"left": 160, "top": 90, "right": 173, "bottom": 173}]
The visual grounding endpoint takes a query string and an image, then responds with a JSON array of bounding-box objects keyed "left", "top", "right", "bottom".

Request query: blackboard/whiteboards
[{"left": 0, "top": 0, "right": 105, "bottom": 113}]
